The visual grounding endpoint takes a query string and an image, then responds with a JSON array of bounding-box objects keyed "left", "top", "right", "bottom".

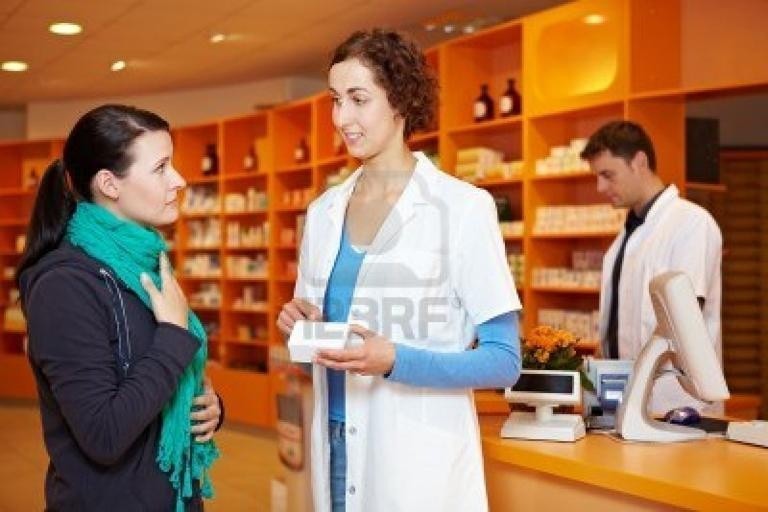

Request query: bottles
[
  {"left": 200, "top": 142, "right": 217, "bottom": 176},
  {"left": 240, "top": 144, "right": 258, "bottom": 172},
  {"left": 469, "top": 82, "right": 495, "bottom": 123},
  {"left": 293, "top": 140, "right": 307, "bottom": 160},
  {"left": 497, "top": 77, "right": 521, "bottom": 117}
]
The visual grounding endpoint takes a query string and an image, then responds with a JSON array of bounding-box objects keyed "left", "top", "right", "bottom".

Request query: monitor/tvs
[{"left": 615, "top": 271, "right": 731, "bottom": 441}]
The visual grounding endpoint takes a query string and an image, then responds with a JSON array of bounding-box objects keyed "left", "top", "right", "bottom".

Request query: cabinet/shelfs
[{"left": 1, "top": 0, "right": 768, "bottom": 429}]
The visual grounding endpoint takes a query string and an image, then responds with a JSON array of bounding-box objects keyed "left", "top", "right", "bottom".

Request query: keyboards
[{"left": 727, "top": 420, "right": 768, "bottom": 448}]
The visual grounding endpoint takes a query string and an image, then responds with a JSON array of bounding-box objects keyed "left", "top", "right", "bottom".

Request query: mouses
[{"left": 665, "top": 408, "right": 699, "bottom": 425}]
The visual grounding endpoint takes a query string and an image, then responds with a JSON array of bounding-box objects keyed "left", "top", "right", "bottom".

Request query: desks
[{"left": 479, "top": 413, "right": 768, "bottom": 512}]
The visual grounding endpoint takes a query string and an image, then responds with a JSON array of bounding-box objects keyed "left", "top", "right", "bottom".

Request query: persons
[
  {"left": 272, "top": 24, "right": 523, "bottom": 510},
  {"left": 581, "top": 119, "right": 725, "bottom": 419},
  {"left": 14, "top": 102, "right": 226, "bottom": 512}
]
